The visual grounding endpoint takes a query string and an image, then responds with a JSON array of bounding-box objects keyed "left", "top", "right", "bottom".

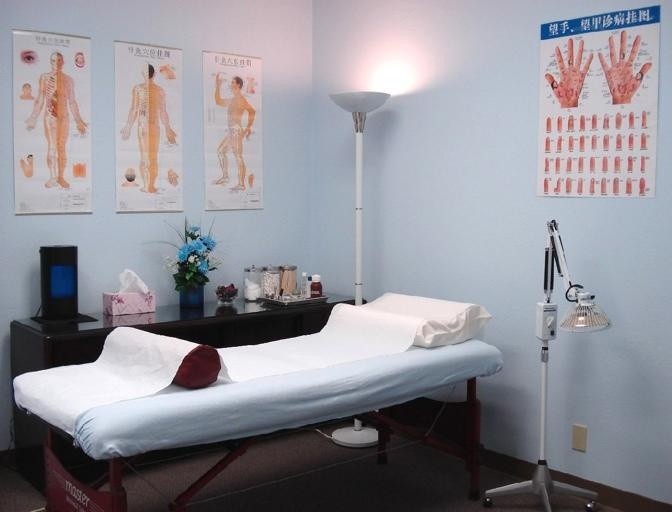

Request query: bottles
[{"left": 242, "top": 261, "right": 324, "bottom": 302}]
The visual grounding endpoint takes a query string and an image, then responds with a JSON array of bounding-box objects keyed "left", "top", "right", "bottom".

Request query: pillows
[{"left": 105, "top": 326, "right": 221, "bottom": 389}]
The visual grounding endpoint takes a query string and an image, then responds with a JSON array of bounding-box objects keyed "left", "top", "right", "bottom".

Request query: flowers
[{"left": 139, "top": 211, "right": 221, "bottom": 297}]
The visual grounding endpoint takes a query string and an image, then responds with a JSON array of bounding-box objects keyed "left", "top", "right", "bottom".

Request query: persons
[
  {"left": 25, "top": 50, "right": 89, "bottom": 190},
  {"left": 19, "top": 154, "right": 34, "bottom": 178},
  {"left": 543, "top": 37, "right": 594, "bottom": 109},
  {"left": 120, "top": 62, "right": 178, "bottom": 196},
  {"left": 19, "top": 50, "right": 38, "bottom": 63},
  {"left": 19, "top": 83, "right": 34, "bottom": 101},
  {"left": 212, "top": 70, "right": 257, "bottom": 192},
  {"left": 167, "top": 169, "right": 179, "bottom": 188},
  {"left": 597, "top": 30, "right": 653, "bottom": 104}
]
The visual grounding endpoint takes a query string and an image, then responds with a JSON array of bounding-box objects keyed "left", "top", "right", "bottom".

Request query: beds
[{"left": 12, "top": 332, "right": 503, "bottom": 512}]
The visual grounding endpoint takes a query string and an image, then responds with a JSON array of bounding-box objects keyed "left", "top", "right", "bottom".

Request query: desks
[{"left": 10, "top": 294, "right": 369, "bottom": 503}]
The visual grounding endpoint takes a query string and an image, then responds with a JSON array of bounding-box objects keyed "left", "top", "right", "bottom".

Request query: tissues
[{"left": 104, "top": 268, "right": 157, "bottom": 318}]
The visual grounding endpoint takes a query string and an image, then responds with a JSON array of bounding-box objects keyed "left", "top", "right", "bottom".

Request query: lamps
[
  {"left": 484, "top": 214, "right": 612, "bottom": 512},
  {"left": 331, "top": 90, "right": 390, "bottom": 447}
]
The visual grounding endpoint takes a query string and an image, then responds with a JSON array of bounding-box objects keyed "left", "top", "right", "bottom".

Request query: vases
[{"left": 179, "top": 283, "right": 205, "bottom": 309}]
